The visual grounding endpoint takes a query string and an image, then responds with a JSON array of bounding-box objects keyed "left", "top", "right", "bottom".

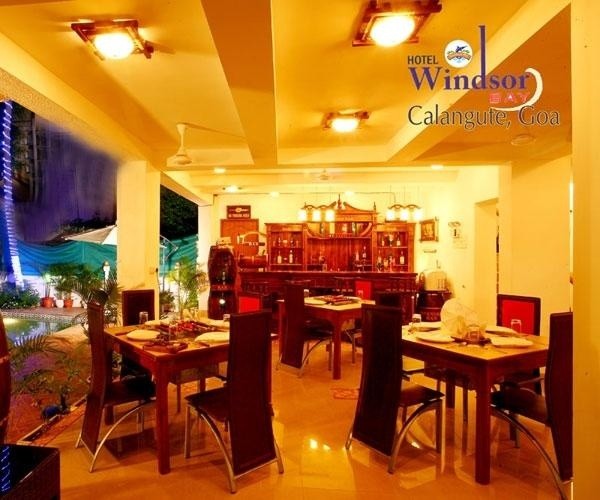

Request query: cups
[
  {"left": 304, "top": 289, "right": 309, "bottom": 298},
  {"left": 168, "top": 324, "right": 178, "bottom": 342},
  {"left": 510, "top": 319, "right": 521, "bottom": 335},
  {"left": 322, "top": 264, "right": 327, "bottom": 272},
  {"left": 138, "top": 312, "right": 149, "bottom": 326},
  {"left": 412, "top": 313, "right": 421, "bottom": 336},
  {"left": 469, "top": 326, "right": 479, "bottom": 347},
  {"left": 223, "top": 313, "right": 230, "bottom": 327}
]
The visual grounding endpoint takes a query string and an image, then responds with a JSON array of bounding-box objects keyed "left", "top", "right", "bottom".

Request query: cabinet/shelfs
[{"left": 239, "top": 202, "right": 418, "bottom": 323}]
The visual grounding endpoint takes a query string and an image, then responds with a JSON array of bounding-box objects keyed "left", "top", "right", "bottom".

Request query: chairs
[
  {"left": 495, "top": 295, "right": 541, "bottom": 394},
  {"left": 276, "top": 286, "right": 331, "bottom": 377},
  {"left": 75, "top": 291, "right": 157, "bottom": 472},
  {"left": 354, "top": 281, "right": 373, "bottom": 300},
  {"left": 185, "top": 312, "right": 285, "bottom": 494},
  {"left": 490, "top": 312, "right": 572, "bottom": 498},
  {"left": 122, "top": 289, "right": 154, "bottom": 325},
  {"left": 346, "top": 304, "right": 444, "bottom": 476}
]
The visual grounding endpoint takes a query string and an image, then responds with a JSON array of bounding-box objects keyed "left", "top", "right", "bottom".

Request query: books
[
  {"left": 143, "top": 341, "right": 188, "bottom": 354},
  {"left": 331, "top": 296, "right": 352, "bottom": 305}
]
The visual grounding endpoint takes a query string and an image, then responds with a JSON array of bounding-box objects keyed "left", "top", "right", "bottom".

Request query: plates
[
  {"left": 486, "top": 324, "right": 514, "bottom": 333},
  {"left": 126, "top": 330, "right": 161, "bottom": 341},
  {"left": 409, "top": 321, "right": 440, "bottom": 331},
  {"left": 452, "top": 335, "right": 491, "bottom": 343},
  {"left": 145, "top": 320, "right": 169, "bottom": 327},
  {"left": 305, "top": 299, "right": 325, "bottom": 305},
  {"left": 195, "top": 331, "right": 230, "bottom": 344},
  {"left": 491, "top": 337, "right": 533, "bottom": 349},
  {"left": 415, "top": 331, "right": 455, "bottom": 343}
]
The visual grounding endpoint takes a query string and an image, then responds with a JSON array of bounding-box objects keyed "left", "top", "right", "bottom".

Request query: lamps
[
  {"left": 385, "top": 184, "right": 424, "bottom": 220},
  {"left": 71, "top": 20, "right": 152, "bottom": 61},
  {"left": 323, "top": 111, "right": 370, "bottom": 132},
  {"left": 353, "top": 0, "right": 442, "bottom": 47},
  {"left": 167, "top": 123, "right": 192, "bottom": 167},
  {"left": 297, "top": 187, "right": 334, "bottom": 222}
]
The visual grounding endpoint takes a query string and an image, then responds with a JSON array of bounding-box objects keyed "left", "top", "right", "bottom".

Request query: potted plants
[{"left": 39, "top": 258, "right": 207, "bottom": 317}]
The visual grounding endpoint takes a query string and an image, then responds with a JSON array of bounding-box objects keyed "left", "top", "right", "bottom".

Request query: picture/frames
[{"left": 419, "top": 219, "right": 435, "bottom": 242}]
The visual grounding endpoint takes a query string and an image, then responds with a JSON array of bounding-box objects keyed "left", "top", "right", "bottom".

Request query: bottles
[
  {"left": 355, "top": 246, "right": 367, "bottom": 262},
  {"left": 377, "top": 231, "right": 405, "bottom": 273},
  {"left": 276, "top": 232, "right": 294, "bottom": 264}
]
